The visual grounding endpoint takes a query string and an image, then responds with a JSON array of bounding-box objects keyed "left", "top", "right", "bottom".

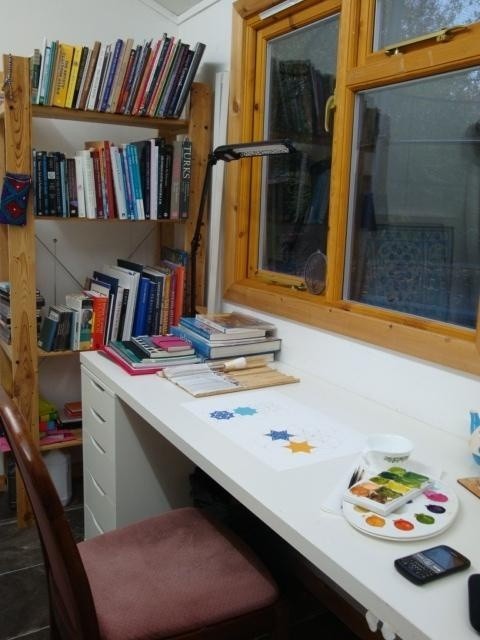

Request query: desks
[{"left": 79, "top": 352, "right": 480, "bottom": 639}]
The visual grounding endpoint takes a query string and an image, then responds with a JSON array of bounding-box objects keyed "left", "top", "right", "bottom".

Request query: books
[
  {"left": 0, "top": 280, "right": 12, "bottom": 346},
  {"left": 268, "top": 56, "right": 379, "bottom": 281}
]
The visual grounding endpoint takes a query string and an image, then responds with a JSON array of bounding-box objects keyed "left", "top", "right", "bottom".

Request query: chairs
[{"left": 0, "top": 387, "right": 277, "bottom": 639}]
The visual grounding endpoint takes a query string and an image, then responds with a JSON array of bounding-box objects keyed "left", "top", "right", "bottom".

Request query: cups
[{"left": 361, "top": 435, "right": 414, "bottom": 477}]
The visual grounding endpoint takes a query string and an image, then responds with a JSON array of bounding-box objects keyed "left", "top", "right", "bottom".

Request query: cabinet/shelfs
[{"left": 0, "top": 54, "right": 214, "bottom": 528}]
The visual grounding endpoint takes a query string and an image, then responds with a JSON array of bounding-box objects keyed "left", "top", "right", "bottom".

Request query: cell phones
[{"left": 395, "top": 544, "right": 470, "bottom": 585}]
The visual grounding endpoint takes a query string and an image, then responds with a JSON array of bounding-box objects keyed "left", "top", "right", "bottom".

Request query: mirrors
[{"left": 304, "top": 253, "right": 327, "bottom": 295}]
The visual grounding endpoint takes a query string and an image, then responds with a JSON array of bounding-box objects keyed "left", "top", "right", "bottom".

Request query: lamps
[{"left": 191, "top": 140, "right": 296, "bottom": 317}]
